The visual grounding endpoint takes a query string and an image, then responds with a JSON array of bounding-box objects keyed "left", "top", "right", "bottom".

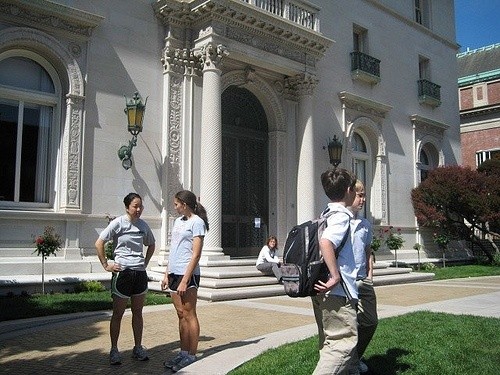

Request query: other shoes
[
  {"left": 358, "top": 361, "right": 368, "bottom": 371},
  {"left": 164, "top": 351, "right": 196, "bottom": 371},
  {"left": 280, "top": 280, "right": 284, "bottom": 285}
]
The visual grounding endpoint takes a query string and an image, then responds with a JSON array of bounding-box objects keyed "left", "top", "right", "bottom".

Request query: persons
[
  {"left": 311, "top": 167, "right": 358, "bottom": 375},
  {"left": 94, "top": 193, "right": 155, "bottom": 365},
  {"left": 160, "top": 190, "right": 209, "bottom": 370},
  {"left": 255, "top": 235, "right": 283, "bottom": 285},
  {"left": 350, "top": 179, "right": 378, "bottom": 375}
]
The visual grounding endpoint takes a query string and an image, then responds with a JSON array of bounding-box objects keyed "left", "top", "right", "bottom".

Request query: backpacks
[{"left": 283, "top": 207, "right": 351, "bottom": 298}]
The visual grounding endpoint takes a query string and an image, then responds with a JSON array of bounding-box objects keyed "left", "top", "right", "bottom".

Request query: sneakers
[
  {"left": 132, "top": 347, "right": 150, "bottom": 361},
  {"left": 110, "top": 347, "right": 123, "bottom": 365}
]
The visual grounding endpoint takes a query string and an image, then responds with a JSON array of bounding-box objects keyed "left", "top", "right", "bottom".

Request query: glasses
[
  {"left": 353, "top": 191, "right": 368, "bottom": 200},
  {"left": 129, "top": 204, "right": 144, "bottom": 210}
]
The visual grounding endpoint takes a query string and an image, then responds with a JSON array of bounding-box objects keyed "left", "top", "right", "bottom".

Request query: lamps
[
  {"left": 118, "top": 91, "right": 150, "bottom": 170},
  {"left": 322, "top": 133, "right": 347, "bottom": 168}
]
